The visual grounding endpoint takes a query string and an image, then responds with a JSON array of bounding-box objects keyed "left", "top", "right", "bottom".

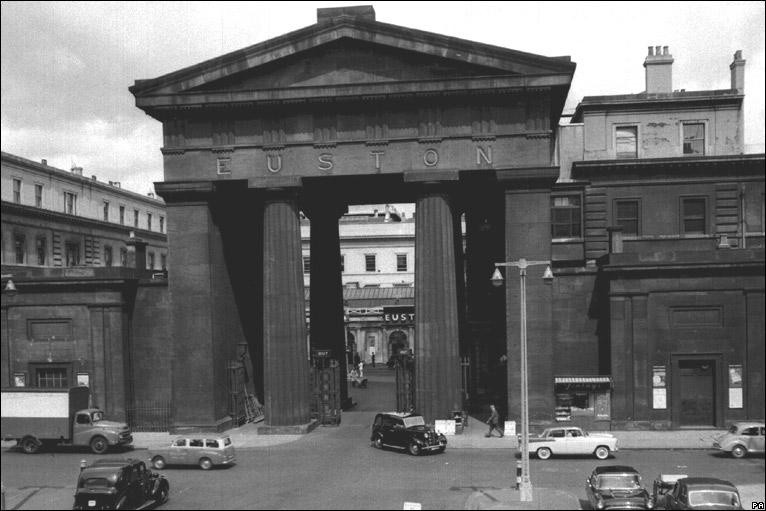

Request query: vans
[{"left": 142, "top": 432, "right": 237, "bottom": 472}]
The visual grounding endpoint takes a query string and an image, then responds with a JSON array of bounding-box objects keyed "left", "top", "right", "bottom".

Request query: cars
[
  {"left": 582, "top": 463, "right": 652, "bottom": 511},
  {"left": 710, "top": 420, "right": 765, "bottom": 458},
  {"left": 663, "top": 474, "right": 746, "bottom": 510},
  {"left": 368, "top": 407, "right": 448, "bottom": 458},
  {"left": 516, "top": 424, "right": 620, "bottom": 460},
  {"left": 70, "top": 457, "right": 171, "bottom": 511}
]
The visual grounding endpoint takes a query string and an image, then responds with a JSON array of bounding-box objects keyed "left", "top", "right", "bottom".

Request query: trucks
[{"left": 0, "top": 383, "right": 136, "bottom": 457}]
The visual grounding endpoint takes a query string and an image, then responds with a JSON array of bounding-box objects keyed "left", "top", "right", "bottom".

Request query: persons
[
  {"left": 350, "top": 351, "right": 375, "bottom": 388},
  {"left": 485, "top": 405, "right": 504, "bottom": 438}
]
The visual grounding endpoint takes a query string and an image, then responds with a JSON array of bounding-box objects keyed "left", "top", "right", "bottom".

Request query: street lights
[{"left": 486, "top": 256, "right": 556, "bottom": 503}]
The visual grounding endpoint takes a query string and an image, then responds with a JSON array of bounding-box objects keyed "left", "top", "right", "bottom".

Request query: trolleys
[{"left": 352, "top": 375, "right": 369, "bottom": 389}]
